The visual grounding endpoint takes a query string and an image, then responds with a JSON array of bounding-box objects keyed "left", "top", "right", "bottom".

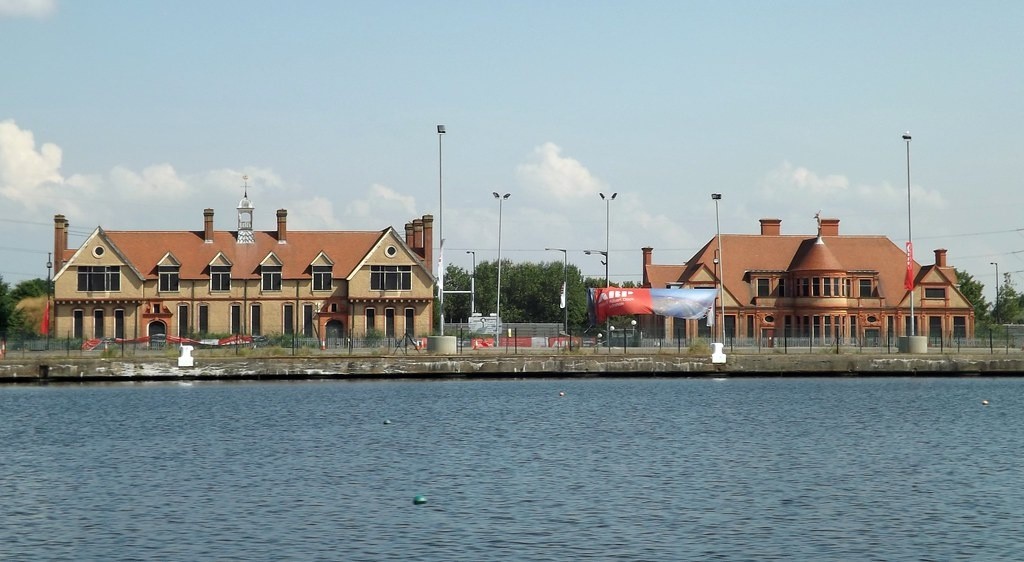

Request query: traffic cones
[
  {"left": 414, "top": 338, "right": 425, "bottom": 350},
  {"left": 1, "top": 340, "right": 6, "bottom": 354},
  {"left": 321, "top": 337, "right": 326, "bottom": 350},
  {"left": 472, "top": 338, "right": 480, "bottom": 350}
]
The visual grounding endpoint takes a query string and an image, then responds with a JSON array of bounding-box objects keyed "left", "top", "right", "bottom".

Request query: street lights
[
  {"left": 467, "top": 251, "right": 474, "bottom": 315},
  {"left": 584, "top": 249, "right": 609, "bottom": 346},
  {"left": 631, "top": 320, "right": 636, "bottom": 338},
  {"left": 991, "top": 262, "right": 998, "bottom": 320},
  {"left": 902, "top": 133, "right": 915, "bottom": 333},
  {"left": 545, "top": 248, "right": 567, "bottom": 337},
  {"left": 437, "top": 124, "right": 446, "bottom": 336},
  {"left": 494, "top": 192, "right": 510, "bottom": 347},
  {"left": 600, "top": 193, "right": 618, "bottom": 348},
  {"left": 711, "top": 193, "right": 726, "bottom": 347}
]
